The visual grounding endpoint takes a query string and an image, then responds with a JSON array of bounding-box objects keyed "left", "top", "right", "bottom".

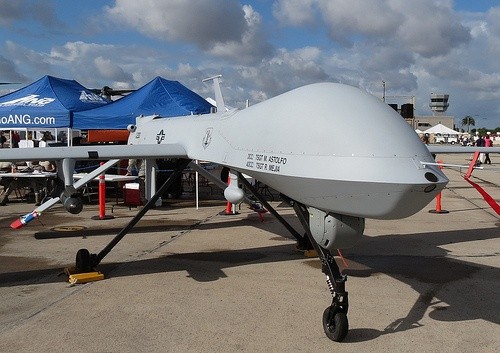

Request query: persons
[
  {"left": 474, "top": 135, "right": 493, "bottom": 164},
  {"left": 125, "top": 159, "right": 158, "bottom": 205},
  {"left": 0, "top": 131, "right": 67, "bottom": 186}
]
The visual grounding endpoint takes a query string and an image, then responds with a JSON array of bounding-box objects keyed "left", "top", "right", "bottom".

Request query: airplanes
[{"left": 0, "top": 74, "right": 500, "bottom": 341}]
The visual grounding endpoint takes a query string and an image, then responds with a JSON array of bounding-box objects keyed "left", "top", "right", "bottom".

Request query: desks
[
  {"left": 73, "top": 173, "right": 137, "bottom": 213},
  {"left": 0, "top": 172, "right": 57, "bottom": 205}
]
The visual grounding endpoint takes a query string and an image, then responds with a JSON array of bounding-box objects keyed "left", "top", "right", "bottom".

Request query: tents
[
  {"left": 71, "top": 77, "right": 217, "bottom": 209},
  {"left": 415, "top": 124, "right": 471, "bottom": 144},
  {"left": 0, "top": 74, "right": 109, "bottom": 172}
]
